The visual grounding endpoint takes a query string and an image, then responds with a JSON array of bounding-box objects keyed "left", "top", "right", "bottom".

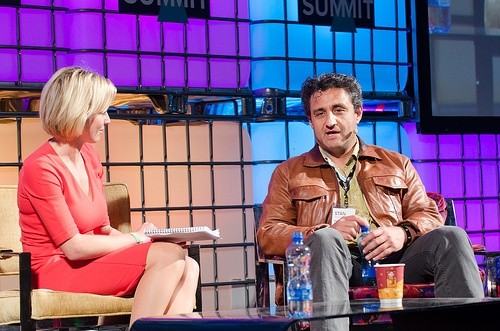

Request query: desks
[{"left": 130, "top": 298, "right": 500, "bottom": 331}]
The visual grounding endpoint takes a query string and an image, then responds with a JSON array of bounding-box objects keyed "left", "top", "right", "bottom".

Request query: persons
[
  {"left": 254, "top": 72, "right": 486, "bottom": 331},
  {"left": 16, "top": 64, "right": 200, "bottom": 331}
]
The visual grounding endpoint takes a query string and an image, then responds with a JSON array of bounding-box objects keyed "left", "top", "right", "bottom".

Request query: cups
[{"left": 374, "top": 264, "right": 406, "bottom": 308}]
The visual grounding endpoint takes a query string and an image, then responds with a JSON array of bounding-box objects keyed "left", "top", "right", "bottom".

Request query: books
[{"left": 144, "top": 226, "right": 220, "bottom": 242}]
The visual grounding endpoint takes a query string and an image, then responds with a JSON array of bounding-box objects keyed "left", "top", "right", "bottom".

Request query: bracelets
[
  {"left": 401, "top": 225, "right": 412, "bottom": 245},
  {"left": 129, "top": 232, "right": 144, "bottom": 245}
]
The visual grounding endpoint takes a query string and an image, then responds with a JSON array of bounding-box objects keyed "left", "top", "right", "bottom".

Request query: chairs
[
  {"left": 0, "top": 182, "right": 202, "bottom": 331},
  {"left": 254, "top": 193, "right": 500, "bottom": 307}
]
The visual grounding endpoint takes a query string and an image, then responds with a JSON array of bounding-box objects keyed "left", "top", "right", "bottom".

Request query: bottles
[
  {"left": 357, "top": 225, "right": 380, "bottom": 286},
  {"left": 286, "top": 232, "right": 313, "bottom": 318}
]
